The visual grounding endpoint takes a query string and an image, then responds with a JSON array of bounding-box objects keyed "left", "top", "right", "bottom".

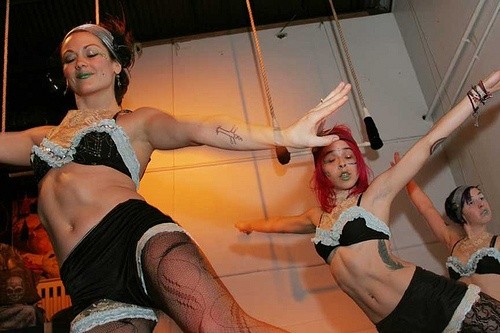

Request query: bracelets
[{"left": 467, "top": 80, "right": 494, "bottom": 127}]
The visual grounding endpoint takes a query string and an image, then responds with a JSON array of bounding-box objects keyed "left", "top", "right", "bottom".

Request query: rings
[{"left": 320, "top": 98, "right": 324, "bottom": 102}]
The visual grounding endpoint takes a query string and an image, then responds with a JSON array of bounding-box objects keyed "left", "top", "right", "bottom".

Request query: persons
[
  {"left": 390, "top": 152, "right": 500, "bottom": 302},
  {"left": 234, "top": 69, "right": 500, "bottom": 333},
  {"left": 0, "top": 277, "right": 37, "bottom": 333},
  {"left": 0, "top": 20, "right": 352, "bottom": 333}
]
